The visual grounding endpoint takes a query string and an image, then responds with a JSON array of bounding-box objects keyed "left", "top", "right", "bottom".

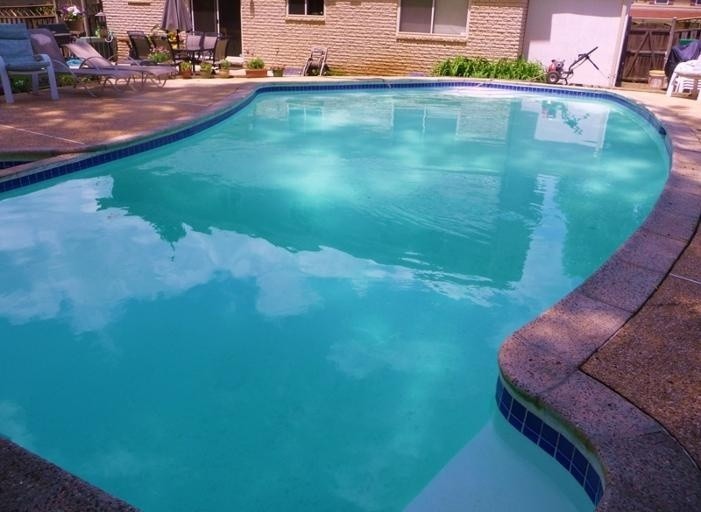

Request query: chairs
[
  {"left": 0, "top": 13, "right": 233, "bottom": 102},
  {"left": 300, "top": 46, "right": 332, "bottom": 76}
]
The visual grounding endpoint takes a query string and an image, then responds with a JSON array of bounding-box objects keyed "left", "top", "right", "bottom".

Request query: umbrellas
[{"left": 158, "top": 1, "right": 194, "bottom": 74}]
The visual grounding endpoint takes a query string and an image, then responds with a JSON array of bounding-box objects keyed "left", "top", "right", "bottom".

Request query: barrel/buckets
[{"left": 648, "top": 70, "right": 666, "bottom": 89}]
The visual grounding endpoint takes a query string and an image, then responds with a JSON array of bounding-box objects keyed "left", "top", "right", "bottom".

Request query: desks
[{"left": 671, "top": 67, "right": 701, "bottom": 100}]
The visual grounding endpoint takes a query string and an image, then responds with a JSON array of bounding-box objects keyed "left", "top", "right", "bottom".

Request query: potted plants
[{"left": 243, "top": 56, "right": 268, "bottom": 79}]
